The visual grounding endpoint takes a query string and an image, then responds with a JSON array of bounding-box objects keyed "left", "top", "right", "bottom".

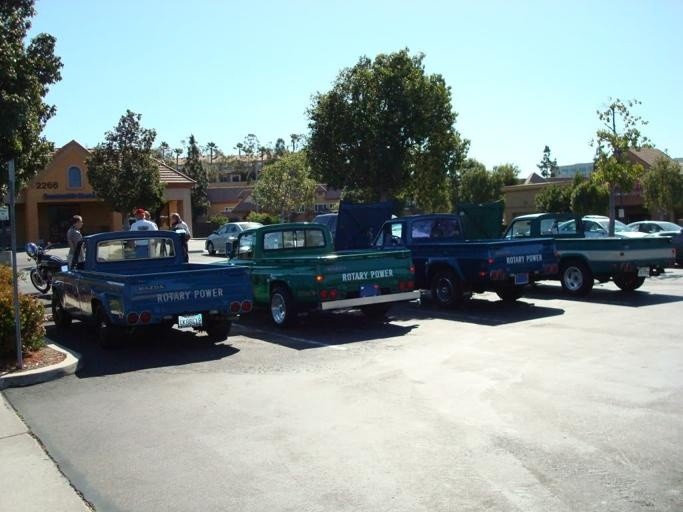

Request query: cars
[
  {"left": 624, "top": 221, "right": 682, "bottom": 265},
  {"left": 557, "top": 216, "right": 651, "bottom": 239},
  {"left": 205, "top": 222, "right": 265, "bottom": 255}
]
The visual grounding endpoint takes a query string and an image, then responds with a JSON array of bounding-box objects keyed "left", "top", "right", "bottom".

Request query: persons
[
  {"left": 66, "top": 214, "right": 85, "bottom": 270},
  {"left": 158, "top": 215, "right": 171, "bottom": 232},
  {"left": 144, "top": 211, "right": 159, "bottom": 259},
  {"left": 129, "top": 208, "right": 159, "bottom": 257},
  {"left": 170, "top": 212, "right": 190, "bottom": 262}
]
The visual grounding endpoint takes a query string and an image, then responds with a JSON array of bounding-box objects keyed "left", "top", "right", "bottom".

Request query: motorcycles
[{"left": 24, "top": 240, "right": 98, "bottom": 293}]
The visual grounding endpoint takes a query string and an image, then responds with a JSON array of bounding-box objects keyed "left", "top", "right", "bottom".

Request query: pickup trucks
[
  {"left": 215, "top": 220, "right": 421, "bottom": 327},
  {"left": 505, "top": 212, "right": 676, "bottom": 299},
  {"left": 367, "top": 214, "right": 559, "bottom": 308},
  {"left": 51, "top": 230, "right": 254, "bottom": 347}
]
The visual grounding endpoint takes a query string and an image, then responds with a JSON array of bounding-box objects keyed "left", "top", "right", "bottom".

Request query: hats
[{"left": 133, "top": 208, "right": 145, "bottom": 216}]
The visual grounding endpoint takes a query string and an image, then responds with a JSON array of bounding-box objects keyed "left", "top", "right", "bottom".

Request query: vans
[{"left": 310, "top": 211, "right": 425, "bottom": 245}]
